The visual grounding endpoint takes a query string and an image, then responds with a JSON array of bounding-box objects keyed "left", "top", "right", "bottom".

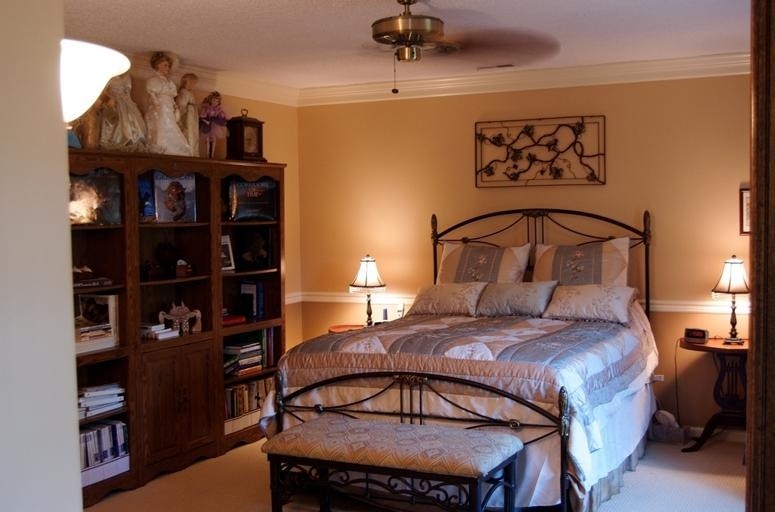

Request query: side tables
[{"left": 679, "top": 338, "right": 749, "bottom": 466}]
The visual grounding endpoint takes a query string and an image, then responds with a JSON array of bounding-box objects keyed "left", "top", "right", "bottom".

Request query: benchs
[{"left": 260, "top": 417, "right": 524, "bottom": 512}]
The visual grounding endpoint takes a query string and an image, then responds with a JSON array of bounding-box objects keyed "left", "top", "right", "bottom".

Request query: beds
[{"left": 260, "top": 209, "right": 664, "bottom": 512}]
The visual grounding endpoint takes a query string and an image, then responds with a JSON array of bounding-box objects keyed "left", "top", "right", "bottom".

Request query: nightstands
[{"left": 328, "top": 326, "right": 364, "bottom": 333}]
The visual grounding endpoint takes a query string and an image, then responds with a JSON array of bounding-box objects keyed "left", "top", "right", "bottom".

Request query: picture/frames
[{"left": 739, "top": 188, "right": 751, "bottom": 235}]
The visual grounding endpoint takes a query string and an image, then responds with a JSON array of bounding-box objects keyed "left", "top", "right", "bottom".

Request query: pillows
[
  {"left": 404, "top": 281, "right": 487, "bottom": 316},
  {"left": 533, "top": 237, "right": 629, "bottom": 286},
  {"left": 541, "top": 283, "right": 638, "bottom": 328},
  {"left": 438, "top": 240, "right": 530, "bottom": 284},
  {"left": 476, "top": 280, "right": 560, "bottom": 317}
]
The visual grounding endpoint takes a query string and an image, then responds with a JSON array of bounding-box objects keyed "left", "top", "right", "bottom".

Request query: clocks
[{"left": 226, "top": 109, "right": 268, "bottom": 162}]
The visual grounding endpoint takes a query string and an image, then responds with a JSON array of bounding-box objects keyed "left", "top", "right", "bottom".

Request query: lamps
[
  {"left": 349, "top": 253, "right": 387, "bottom": 327},
  {"left": 60, "top": 39, "right": 130, "bottom": 129},
  {"left": 371, "top": 0, "right": 444, "bottom": 94},
  {"left": 713, "top": 254, "right": 751, "bottom": 344}
]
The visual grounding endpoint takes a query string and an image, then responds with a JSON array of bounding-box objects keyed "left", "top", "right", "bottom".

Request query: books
[
  {"left": 74, "top": 292, "right": 130, "bottom": 473},
  {"left": 224, "top": 274, "right": 283, "bottom": 422}
]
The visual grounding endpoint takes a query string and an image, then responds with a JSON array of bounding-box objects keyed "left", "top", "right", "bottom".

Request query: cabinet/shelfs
[{"left": 69, "top": 148, "right": 287, "bottom": 509}]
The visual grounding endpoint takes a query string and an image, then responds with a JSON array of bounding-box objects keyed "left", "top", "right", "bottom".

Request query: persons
[
  {"left": 146, "top": 53, "right": 191, "bottom": 154},
  {"left": 176, "top": 70, "right": 201, "bottom": 156},
  {"left": 97, "top": 72, "right": 146, "bottom": 152},
  {"left": 198, "top": 91, "right": 227, "bottom": 158}
]
